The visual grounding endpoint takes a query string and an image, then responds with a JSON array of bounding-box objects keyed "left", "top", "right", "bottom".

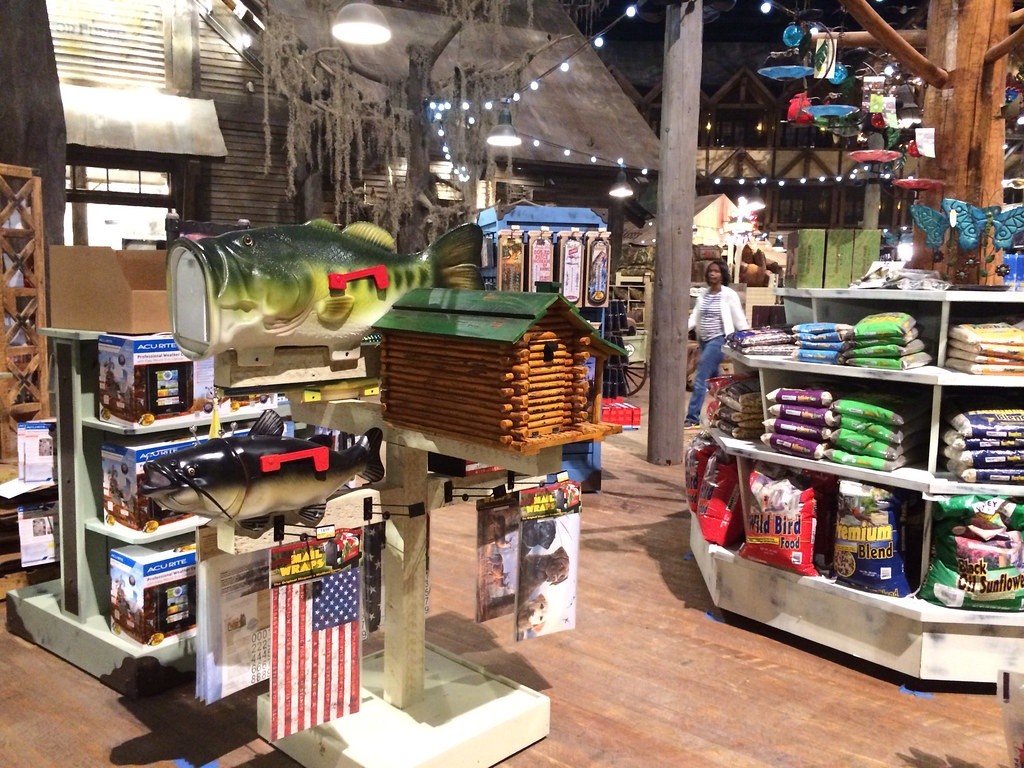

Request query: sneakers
[{"left": 684, "top": 421, "right": 701, "bottom": 430}]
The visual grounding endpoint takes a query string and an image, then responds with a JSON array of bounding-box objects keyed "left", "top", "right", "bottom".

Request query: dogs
[{"left": 517, "top": 518, "right": 569, "bottom": 632}]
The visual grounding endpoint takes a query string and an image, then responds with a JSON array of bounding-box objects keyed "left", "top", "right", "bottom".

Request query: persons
[{"left": 683, "top": 257, "right": 752, "bottom": 431}]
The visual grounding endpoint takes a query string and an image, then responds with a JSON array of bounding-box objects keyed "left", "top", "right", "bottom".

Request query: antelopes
[{"left": 478, "top": 514, "right": 514, "bottom": 588}]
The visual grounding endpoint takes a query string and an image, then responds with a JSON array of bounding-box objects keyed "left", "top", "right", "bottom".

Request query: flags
[{"left": 270, "top": 564, "right": 362, "bottom": 743}]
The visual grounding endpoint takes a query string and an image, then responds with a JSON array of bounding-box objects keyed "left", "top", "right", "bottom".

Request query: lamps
[
  {"left": 609, "top": 165, "right": 634, "bottom": 199},
  {"left": 487, "top": 100, "right": 522, "bottom": 148},
  {"left": 331, "top": 0, "right": 392, "bottom": 45}
]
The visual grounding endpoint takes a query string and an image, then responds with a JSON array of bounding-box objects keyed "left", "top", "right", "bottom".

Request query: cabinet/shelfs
[
  {"left": 6, "top": 323, "right": 385, "bottom": 702},
  {"left": 688, "top": 288, "right": 1024, "bottom": 682}
]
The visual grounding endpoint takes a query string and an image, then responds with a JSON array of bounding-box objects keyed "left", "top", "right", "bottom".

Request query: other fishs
[
  {"left": 168, "top": 219, "right": 484, "bottom": 360},
  {"left": 138, "top": 409, "right": 386, "bottom": 532}
]
